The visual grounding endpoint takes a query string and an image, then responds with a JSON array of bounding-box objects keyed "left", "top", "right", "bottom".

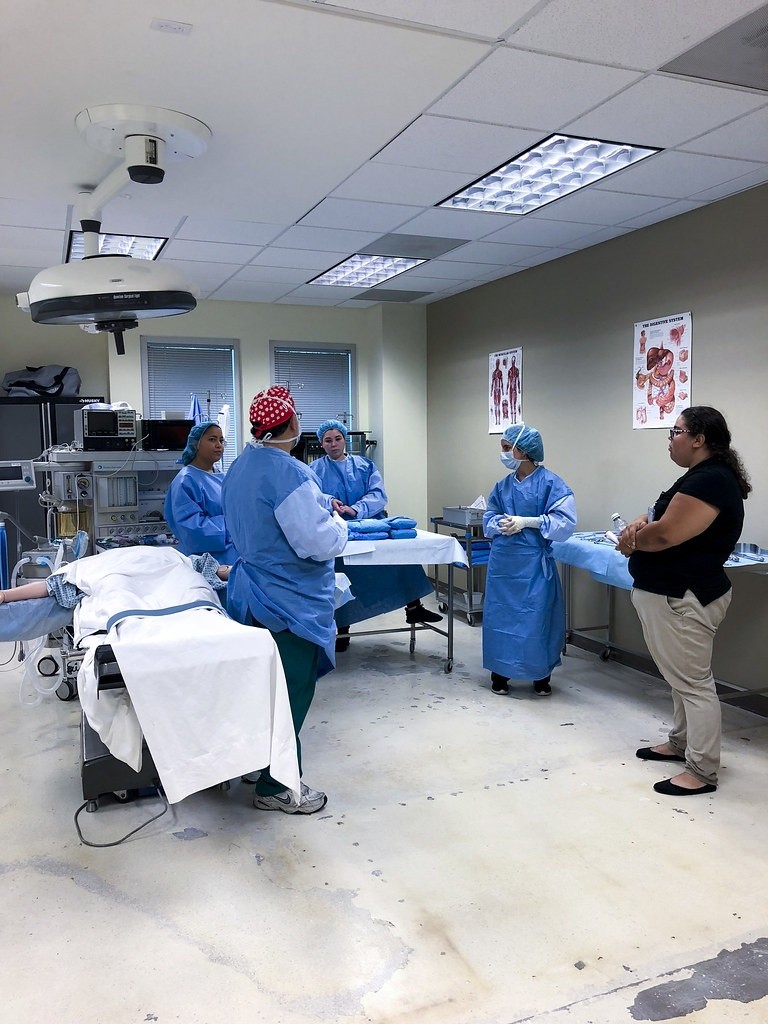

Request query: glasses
[
  {"left": 295, "top": 411, "right": 303, "bottom": 420},
  {"left": 669, "top": 429, "right": 693, "bottom": 440}
]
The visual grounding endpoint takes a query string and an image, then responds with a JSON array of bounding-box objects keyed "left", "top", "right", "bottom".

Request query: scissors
[{"left": 575, "top": 534, "right": 595, "bottom": 539}]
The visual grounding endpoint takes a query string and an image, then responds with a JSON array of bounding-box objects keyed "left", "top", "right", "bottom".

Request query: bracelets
[{"left": 1, "top": 590, "right": 6, "bottom": 602}]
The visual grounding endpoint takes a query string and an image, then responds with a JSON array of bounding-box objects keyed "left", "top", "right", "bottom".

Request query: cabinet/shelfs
[{"left": 430, "top": 516, "right": 495, "bottom": 626}]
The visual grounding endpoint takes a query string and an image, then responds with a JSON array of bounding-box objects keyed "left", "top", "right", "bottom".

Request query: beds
[{"left": 69, "top": 599, "right": 302, "bottom": 805}]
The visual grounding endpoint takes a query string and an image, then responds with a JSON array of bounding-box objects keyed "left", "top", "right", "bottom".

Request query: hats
[{"left": 250, "top": 385, "right": 295, "bottom": 437}]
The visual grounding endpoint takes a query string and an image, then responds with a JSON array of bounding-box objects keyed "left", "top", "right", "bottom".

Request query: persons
[
  {"left": 310, "top": 420, "right": 442, "bottom": 652},
  {"left": 615, "top": 406, "right": 752, "bottom": 796},
  {"left": 0, "top": 547, "right": 234, "bottom": 606},
  {"left": 481, "top": 425, "right": 575, "bottom": 696},
  {"left": 221, "top": 385, "right": 350, "bottom": 814},
  {"left": 163, "top": 420, "right": 241, "bottom": 568}
]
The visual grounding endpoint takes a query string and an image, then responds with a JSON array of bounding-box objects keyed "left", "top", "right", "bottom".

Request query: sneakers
[
  {"left": 241, "top": 771, "right": 262, "bottom": 784},
  {"left": 253, "top": 781, "right": 327, "bottom": 815}
]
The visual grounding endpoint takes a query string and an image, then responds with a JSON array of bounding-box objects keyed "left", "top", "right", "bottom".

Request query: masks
[
  {"left": 499, "top": 425, "right": 529, "bottom": 471},
  {"left": 250, "top": 389, "right": 302, "bottom": 448}
]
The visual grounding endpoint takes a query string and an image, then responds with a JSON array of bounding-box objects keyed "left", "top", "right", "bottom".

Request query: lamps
[{"left": 13, "top": 103, "right": 212, "bottom": 352}]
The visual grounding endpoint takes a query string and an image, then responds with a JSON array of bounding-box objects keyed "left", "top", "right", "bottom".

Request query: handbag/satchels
[{"left": 2, "top": 365, "right": 81, "bottom": 397}]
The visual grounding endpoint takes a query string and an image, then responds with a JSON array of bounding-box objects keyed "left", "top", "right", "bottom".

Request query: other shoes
[
  {"left": 492, "top": 682, "right": 509, "bottom": 694},
  {"left": 654, "top": 778, "right": 717, "bottom": 796},
  {"left": 334, "top": 634, "right": 350, "bottom": 652},
  {"left": 406, "top": 604, "right": 443, "bottom": 624},
  {"left": 636, "top": 747, "right": 687, "bottom": 762},
  {"left": 532, "top": 682, "right": 552, "bottom": 696}
]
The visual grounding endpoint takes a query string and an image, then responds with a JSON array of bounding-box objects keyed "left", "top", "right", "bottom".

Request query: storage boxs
[{"left": 443, "top": 505, "right": 485, "bottom": 524}]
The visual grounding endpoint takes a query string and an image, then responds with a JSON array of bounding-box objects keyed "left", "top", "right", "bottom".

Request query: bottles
[{"left": 611, "top": 513, "right": 629, "bottom": 536}]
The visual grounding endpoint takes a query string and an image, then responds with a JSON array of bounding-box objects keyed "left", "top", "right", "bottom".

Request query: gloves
[{"left": 499, "top": 513, "right": 540, "bottom": 536}]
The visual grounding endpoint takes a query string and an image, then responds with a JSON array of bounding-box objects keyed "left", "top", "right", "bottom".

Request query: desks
[
  {"left": 333, "top": 527, "right": 459, "bottom": 671},
  {"left": 550, "top": 531, "right": 664, "bottom": 679}
]
